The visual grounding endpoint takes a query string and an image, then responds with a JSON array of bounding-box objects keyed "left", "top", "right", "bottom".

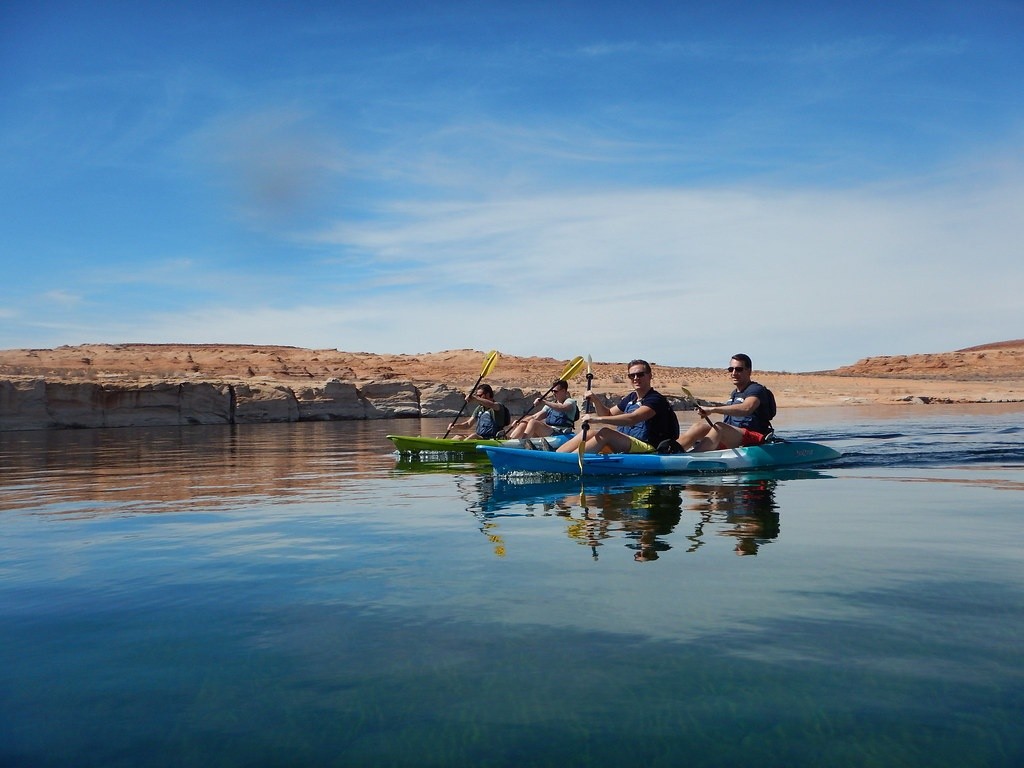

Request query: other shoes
[
  {"left": 541, "top": 437, "right": 556, "bottom": 452},
  {"left": 525, "top": 440, "right": 538, "bottom": 450}
]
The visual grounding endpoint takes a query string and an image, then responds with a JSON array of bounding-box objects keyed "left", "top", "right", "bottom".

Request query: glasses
[
  {"left": 477, "top": 392, "right": 486, "bottom": 398},
  {"left": 551, "top": 389, "right": 562, "bottom": 393},
  {"left": 728, "top": 367, "right": 744, "bottom": 373},
  {"left": 628, "top": 372, "right": 647, "bottom": 380}
]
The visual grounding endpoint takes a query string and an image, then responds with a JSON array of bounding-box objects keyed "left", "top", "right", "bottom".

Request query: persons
[
  {"left": 446, "top": 384, "right": 505, "bottom": 440},
  {"left": 523, "top": 359, "right": 670, "bottom": 454},
  {"left": 676, "top": 353, "right": 774, "bottom": 454},
  {"left": 494, "top": 378, "right": 576, "bottom": 439}
]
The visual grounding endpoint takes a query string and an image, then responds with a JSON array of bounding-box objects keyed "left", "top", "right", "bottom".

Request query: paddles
[
  {"left": 442, "top": 349, "right": 498, "bottom": 439},
  {"left": 577, "top": 353, "right": 594, "bottom": 475},
  {"left": 505, "top": 355, "right": 585, "bottom": 434},
  {"left": 681, "top": 386, "right": 714, "bottom": 427}
]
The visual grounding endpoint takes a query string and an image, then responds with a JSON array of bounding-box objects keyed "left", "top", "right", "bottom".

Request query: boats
[
  {"left": 476, "top": 426, "right": 844, "bottom": 478},
  {"left": 386, "top": 434, "right": 542, "bottom": 473},
  {"left": 477, "top": 466, "right": 840, "bottom": 524}
]
